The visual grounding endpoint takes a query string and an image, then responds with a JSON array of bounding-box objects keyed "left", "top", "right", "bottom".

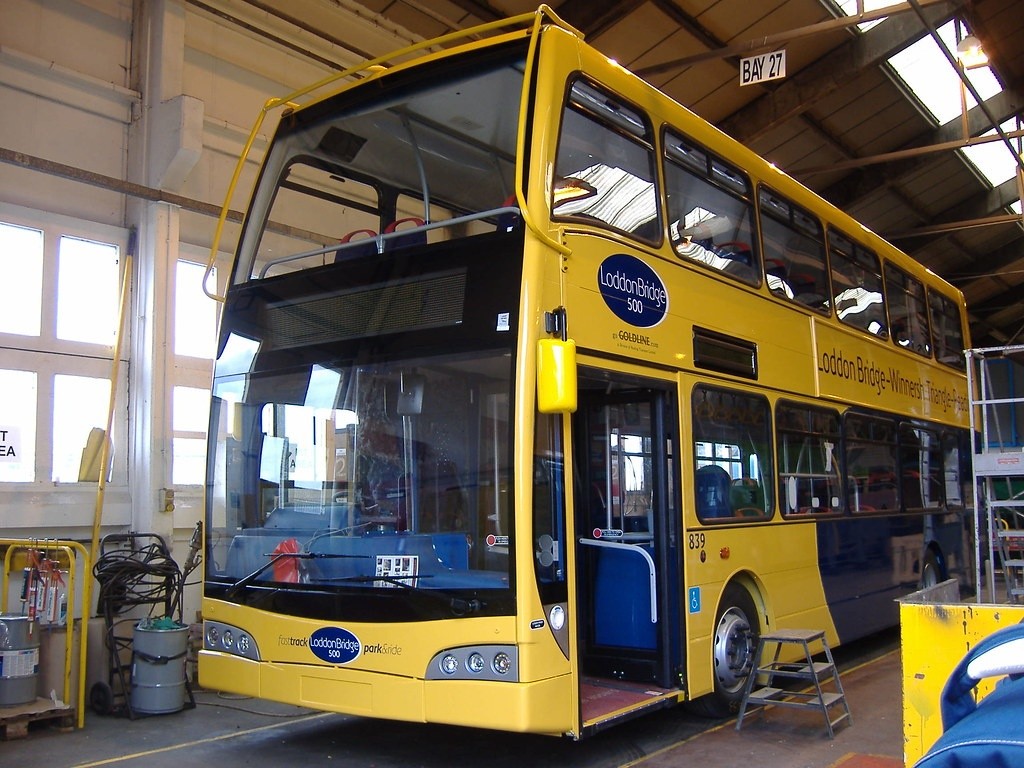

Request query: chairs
[
  {"left": 334, "top": 229, "right": 378, "bottom": 263},
  {"left": 383, "top": 218, "right": 427, "bottom": 252},
  {"left": 787, "top": 274, "right": 816, "bottom": 294},
  {"left": 902, "top": 470, "right": 922, "bottom": 508},
  {"left": 714, "top": 242, "right": 753, "bottom": 266},
  {"left": 863, "top": 471, "right": 896, "bottom": 493},
  {"left": 765, "top": 258, "right": 787, "bottom": 283},
  {"left": 731, "top": 478, "right": 761, "bottom": 515}
]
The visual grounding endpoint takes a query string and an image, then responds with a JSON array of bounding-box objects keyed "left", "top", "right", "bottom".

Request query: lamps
[{"left": 956, "top": 34, "right": 991, "bottom": 70}]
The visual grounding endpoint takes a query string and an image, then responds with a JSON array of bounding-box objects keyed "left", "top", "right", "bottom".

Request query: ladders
[
  {"left": 735, "top": 627, "right": 853, "bottom": 736},
  {"left": 964, "top": 346, "right": 1024, "bottom": 603}
]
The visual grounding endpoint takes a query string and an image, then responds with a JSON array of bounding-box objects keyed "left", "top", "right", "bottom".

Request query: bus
[{"left": 195, "top": 4, "right": 982, "bottom": 742}]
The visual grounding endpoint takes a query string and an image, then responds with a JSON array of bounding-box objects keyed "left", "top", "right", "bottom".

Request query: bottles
[{"left": 59, "top": 594, "right": 67, "bottom": 626}]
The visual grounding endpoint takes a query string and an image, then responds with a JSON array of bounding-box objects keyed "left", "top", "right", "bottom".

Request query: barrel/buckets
[
  {"left": 0, "top": 612, "right": 42, "bottom": 709},
  {"left": 187, "top": 623, "right": 203, "bottom": 682},
  {"left": 130, "top": 620, "right": 189, "bottom": 714}
]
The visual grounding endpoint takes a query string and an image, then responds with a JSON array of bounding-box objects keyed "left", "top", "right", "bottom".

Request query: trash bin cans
[{"left": 130, "top": 622, "right": 190, "bottom": 716}]
